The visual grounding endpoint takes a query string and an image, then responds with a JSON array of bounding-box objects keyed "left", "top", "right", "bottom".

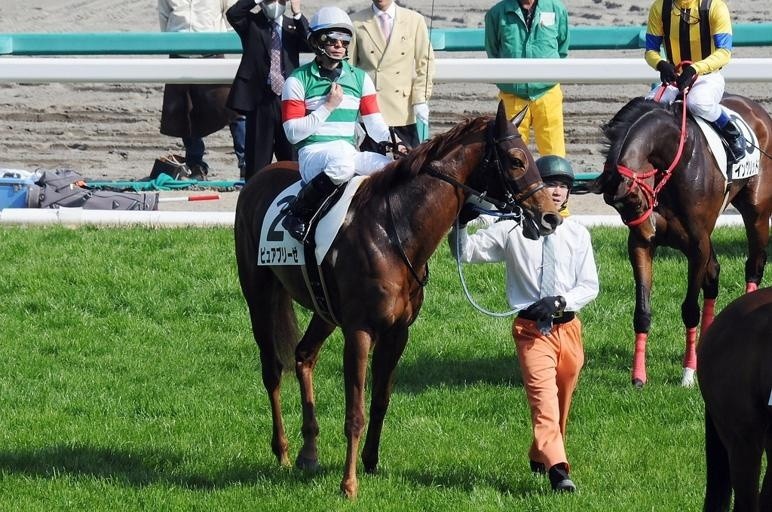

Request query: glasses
[
  {"left": 671, "top": 1, "right": 700, "bottom": 25},
  {"left": 326, "top": 31, "right": 350, "bottom": 46}
]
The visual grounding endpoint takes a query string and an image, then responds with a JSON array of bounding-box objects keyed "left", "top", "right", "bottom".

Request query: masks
[{"left": 261, "top": 2, "right": 286, "bottom": 19}]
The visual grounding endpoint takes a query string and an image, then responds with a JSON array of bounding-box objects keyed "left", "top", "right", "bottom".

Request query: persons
[
  {"left": 279, "top": 4, "right": 410, "bottom": 242},
  {"left": 346, "top": 0, "right": 436, "bottom": 152},
  {"left": 643, "top": 0, "right": 747, "bottom": 155},
  {"left": 225, "top": 0, "right": 316, "bottom": 184},
  {"left": 445, "top": 153, "right": 601, "bottom": 490},
  {"left": 158, "top": 0, "right": 247, "bottom": 181},
  {"left": 484, "top": 0, "right": 570, "bottom": 216}
]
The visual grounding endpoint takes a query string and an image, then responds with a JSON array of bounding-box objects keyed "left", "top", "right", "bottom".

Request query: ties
[
  {"left": 270, "top": 22, "right": 285, "bottom": 96},
  {"left": 380, "top": 13, "right": 391, "bottom": 44}
]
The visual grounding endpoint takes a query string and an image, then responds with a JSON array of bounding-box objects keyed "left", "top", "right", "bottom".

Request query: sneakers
[
  {"left": 530, "top": 458, "right": 576, "bottom": 492},
  {"left": 189, "top": 172, "right": 207, "bottom": 181}
]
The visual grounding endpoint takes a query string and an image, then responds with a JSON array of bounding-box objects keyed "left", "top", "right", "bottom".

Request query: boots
[
  {"left": 281, "top": 172, "right": 339, "bottom": 242},
  {"left": 713, "top": 109, "right": 745, "bottom": 161}
]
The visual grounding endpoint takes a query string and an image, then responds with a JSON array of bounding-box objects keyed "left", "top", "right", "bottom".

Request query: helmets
[
  {"left": 307, "top": 7, "right": 353, "bottom": 40},
  {"left": 535, "top": 156, "right": 574, "bottom": 188}
]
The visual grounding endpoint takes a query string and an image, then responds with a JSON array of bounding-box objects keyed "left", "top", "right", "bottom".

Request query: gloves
[
  {"left": 413, "top": 103, "right": 429, "bottom": 121},
  {"left": 677, "top": 66, "right": 695, "bottom": 91},
  {"left": 656, "top": 61, "right": 677, "bottom": 85},
  {"left": 527, "top": 295, "right": 566, "bottom": 320}
]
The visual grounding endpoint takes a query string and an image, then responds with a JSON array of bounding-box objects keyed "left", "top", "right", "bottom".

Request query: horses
[
  {"left": 569, "top": 91, "right": 772, "bottom": 391},
  {"left": 694, "top": 285, "right": 772, "bottom": 512},
  {"left": 234, "top": 97, "right": 564, "bottom": 500}
]
